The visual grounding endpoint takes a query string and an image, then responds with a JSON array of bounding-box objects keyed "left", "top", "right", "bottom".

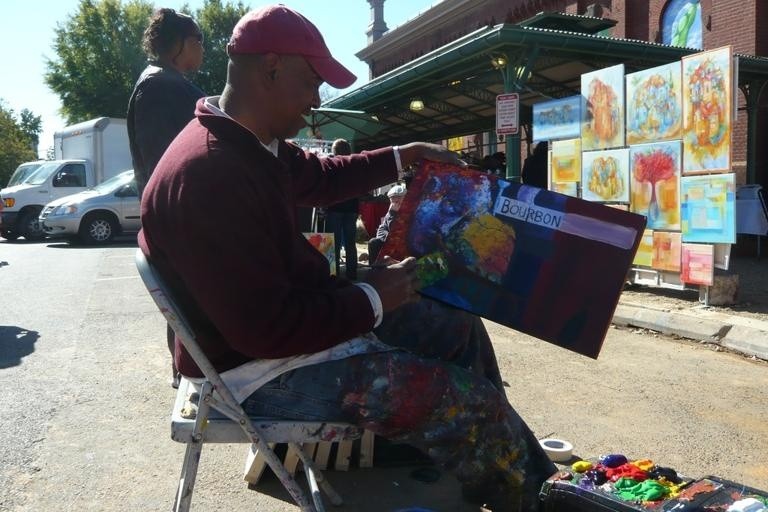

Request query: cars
[{"left": 38, "top": 169, "right": 143, "bottom": 243}]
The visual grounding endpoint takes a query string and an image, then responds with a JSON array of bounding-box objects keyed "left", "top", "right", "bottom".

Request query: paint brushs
[{"left": 414, "top": 264, "right": 440, "bottom": 270}]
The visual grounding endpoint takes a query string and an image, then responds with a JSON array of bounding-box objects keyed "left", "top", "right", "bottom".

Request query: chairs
[{"left": 133, "top": 250, "right": 366, "bottom": 511}]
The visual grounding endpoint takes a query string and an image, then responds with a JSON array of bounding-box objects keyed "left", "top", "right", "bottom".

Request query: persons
[
  {"left": 135, "top": 3, "right": 560, "bottom": 509},
  {"left": 125, "top": 2, "right": 211, "bottom": 389},
  {"left": 457, "top": 140, "right": 547, "bottom": 191},
  {"left": 281, "top": 137, "right": 421, "bottom": 283}
]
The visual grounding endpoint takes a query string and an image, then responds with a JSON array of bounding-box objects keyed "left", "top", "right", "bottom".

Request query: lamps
[{"left": 409, "top": 95, "right": 425, "bottom": 112}]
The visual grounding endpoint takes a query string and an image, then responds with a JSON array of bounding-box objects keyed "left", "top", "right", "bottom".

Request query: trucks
[{"left": 0, "top": 117, "right": 142, "bottom": 243}]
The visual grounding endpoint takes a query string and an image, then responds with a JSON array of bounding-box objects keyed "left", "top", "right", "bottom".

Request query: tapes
[{"left": 538, "top": 438, "right": 573, "bottom": 462}]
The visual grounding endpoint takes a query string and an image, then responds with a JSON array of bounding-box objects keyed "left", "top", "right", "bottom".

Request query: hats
[{"left": 226, "top": 2, "right": 359, "bottom": 89}]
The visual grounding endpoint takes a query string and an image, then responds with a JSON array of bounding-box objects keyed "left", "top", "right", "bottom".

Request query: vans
[{"left": 6, "top": 160, "right": 46, "bottom": 188}]
